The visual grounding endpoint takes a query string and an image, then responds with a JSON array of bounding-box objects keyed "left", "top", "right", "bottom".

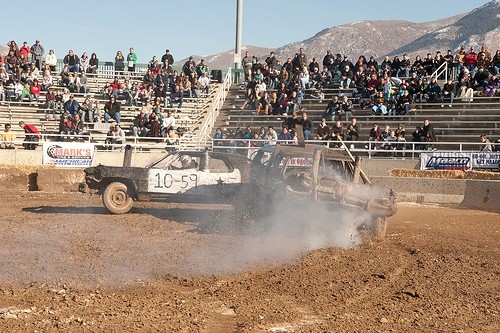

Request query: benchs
[{"left": 0, "top": 70, "right": 500, "bottom": 159}]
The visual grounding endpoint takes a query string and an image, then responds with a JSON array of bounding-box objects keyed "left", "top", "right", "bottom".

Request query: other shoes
[
  {"left": 382, "top": 118, "right": 384, "bottom": 119},
  {"left": 400, "top": 117, "right": 403, "bottom": 120},
  {"left": 256, "top": 112, "right": 259, "bottom": 115},
  {"left": 265, "top": 113, "right": 268, "bottom": 114},
  {"left": 240, "top": 107, "right": 244, "bottom": 109},
  {"left": 393, "top": 118, "right": 395, "bottom": 120}
]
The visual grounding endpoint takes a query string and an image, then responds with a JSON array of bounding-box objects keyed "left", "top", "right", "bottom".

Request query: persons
[
  {"left": 479, "top": 134, "right": 492, "bottom": 152},
  {"left": 0, "top": 38, "right": 99, "bottom": 106},
  {"left": 19, "top": 121, "right": 41, "bottom": 149},
  {"left": 368, "top": 120, "right": 436, "bottom": 156},
  {"left": 115, "top": 50, "right": 124, "bottom": 77},
  {"left": 127, "top": 47, "right": 137, "bottom": 72},
  {"left": 242, "top": 46, "right": 500, "bottom": 118},
  {"left": 60, "top": 94, "right": 177, "bottom": 153},
  {"left": 102, "top": 50, "right": 210, "bottom": 107},
  {"left": 180, "top": 155, "right": 198, "bottom": 168},
  {"left": 214, "top": 112, "right": 357, "bottom": 151},
  {"left": 0, "top": 124, "right": 14, "bottom": 149}
]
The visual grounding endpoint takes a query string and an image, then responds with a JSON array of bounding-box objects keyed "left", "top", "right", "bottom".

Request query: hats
[
  {"left": 166, "top": 49, "right": 169, "bottom": 52},
  {"left": 36, "top": 40, "right": 40, "bottom": 43}
]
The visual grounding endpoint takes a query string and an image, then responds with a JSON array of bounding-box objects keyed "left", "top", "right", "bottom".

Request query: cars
[
  {"left": 84, "top": 150, "right": 251, "bottom": 214},
  {"left": 235, "top": 144, "right": 397, "bottom": 242}
]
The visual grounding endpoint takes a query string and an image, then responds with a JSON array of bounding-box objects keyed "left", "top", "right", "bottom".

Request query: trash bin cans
[{"left": 211, "top": 70, "right": 221, "bottom": 82}]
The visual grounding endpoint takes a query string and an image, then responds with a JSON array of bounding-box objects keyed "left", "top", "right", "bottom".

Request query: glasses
[
  {"left": 7, "top": 127, "right": 10, "bottom": 128},
  {"left": 70, "top": 97, "right": 74, "bottom": 98}
]
